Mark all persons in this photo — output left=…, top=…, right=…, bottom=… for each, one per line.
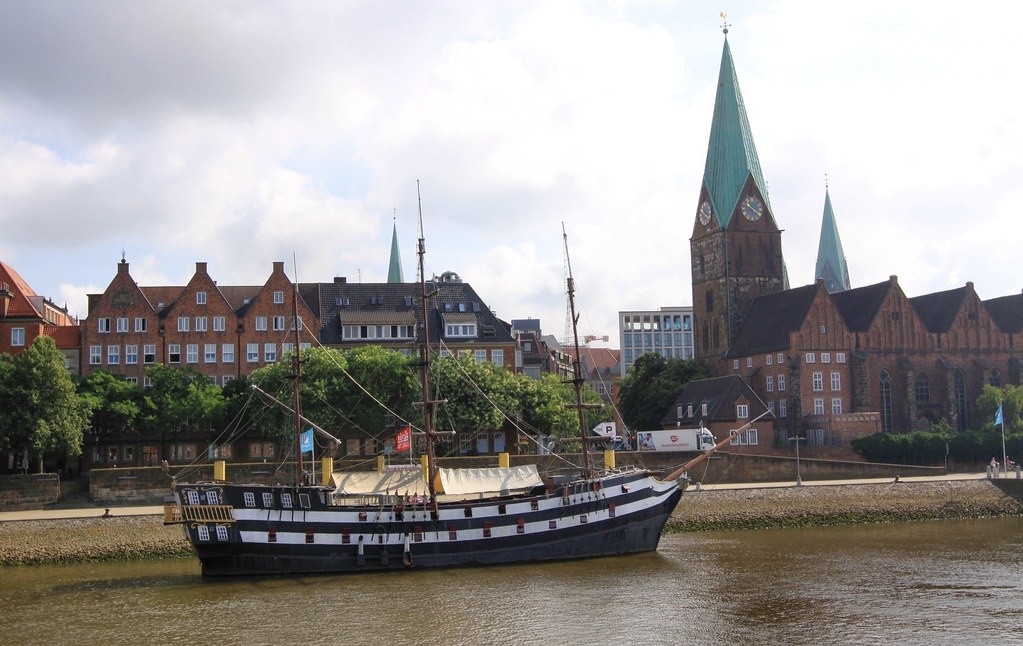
left=594, top=440, right=605, bottom=452
left=1003, top=456, right=1012, bottom=470
left=641, top=437, right=655, bottom=449
left=554, top=440, right=567, bottom=454
left=990, top=458, right=998, bottom=471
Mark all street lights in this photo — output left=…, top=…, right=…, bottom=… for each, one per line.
left=788, top=435, right=806, bottom=485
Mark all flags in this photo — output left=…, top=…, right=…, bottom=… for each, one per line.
left=300, top=428, right=313, bottom=452
left=396, top=428, right=410, bottom=451
left=994, top=405, right=1002, bottom=426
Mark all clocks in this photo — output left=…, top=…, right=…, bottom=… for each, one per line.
left=742, top=196, right=763, bottom=221
left=698, top=202, right=712, bottom=225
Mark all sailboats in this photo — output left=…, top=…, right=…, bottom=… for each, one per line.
left=164, top=178, right=771, bottom=579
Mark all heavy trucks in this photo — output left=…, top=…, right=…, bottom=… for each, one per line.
left=636, top=427, right=716, bottom=452
